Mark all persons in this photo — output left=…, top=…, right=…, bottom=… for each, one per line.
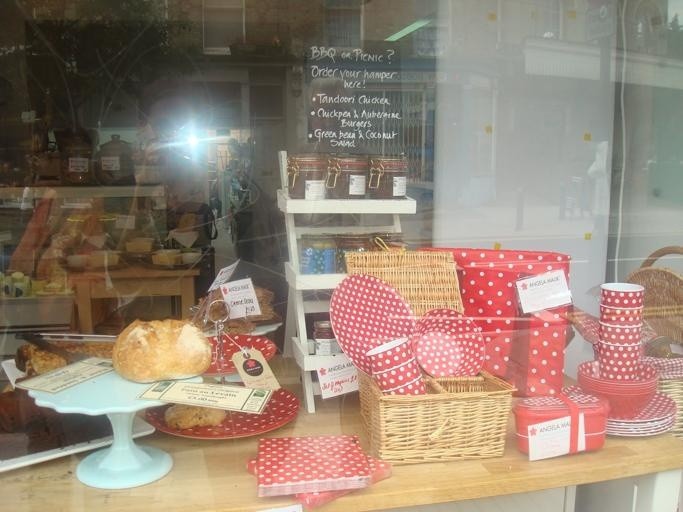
left=288, top=75, right=384, bottom=229
left=102, top=75, right=255, bottom=300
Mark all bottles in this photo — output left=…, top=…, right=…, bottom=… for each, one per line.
left=300, top=232, right=408, bottom=274
left=287, top=154, right=409, bottom=200
left=63, top=136, right=96, bottom=185
left=94, top=134, right=136, bottom=186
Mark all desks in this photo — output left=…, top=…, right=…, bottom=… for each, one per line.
left=2, top=324, right=683, bottom=510
left=72, top=260, right=204, bottom=338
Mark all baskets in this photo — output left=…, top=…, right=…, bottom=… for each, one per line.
left=356, top=367, right=518, bottom=467
left=623, top=245, right=682, bottom=347
left=640, top=356, right=682, bottom=438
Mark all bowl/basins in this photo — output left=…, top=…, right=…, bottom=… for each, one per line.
left=411, top=309, right=487, bottom=381
left=575, top=356, right=664, bottom=419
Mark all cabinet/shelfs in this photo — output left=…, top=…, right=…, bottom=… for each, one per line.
left=275, top=145, right=417, bottom=420
left=2, top=182, right=168, bottom=301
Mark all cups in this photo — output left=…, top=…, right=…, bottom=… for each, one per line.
left=364, top=337, right=427, bottom=398
left=593, top=280, right=648, bottom=385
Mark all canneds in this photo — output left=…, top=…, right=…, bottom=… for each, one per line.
left=301, top=232, right=407, bottom=275
left=312, top=321, right=343, bottom=355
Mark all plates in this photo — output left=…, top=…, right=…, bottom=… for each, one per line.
left=559, top=387, right=677, bottom=438
left=328, top=273, right=418, bottom=379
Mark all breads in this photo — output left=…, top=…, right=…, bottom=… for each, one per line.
left=15, top=285, right=274, bottom=430
